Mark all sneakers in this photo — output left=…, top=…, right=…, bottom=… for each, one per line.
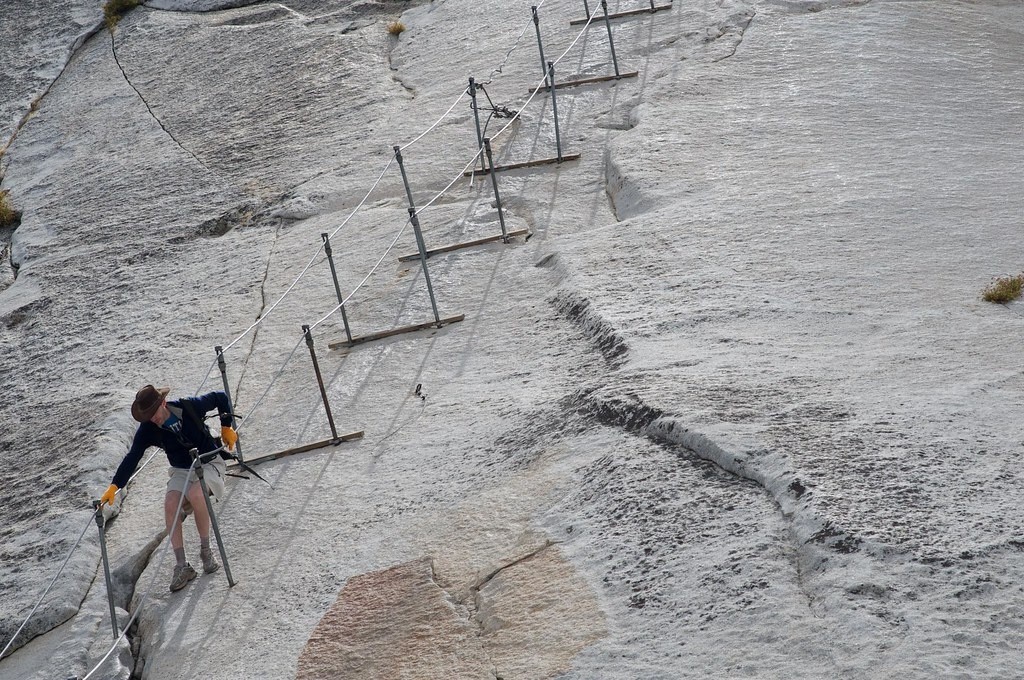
left=170, top=561, right=197, bottom=592
left=199, top=546, right=220, bottom=574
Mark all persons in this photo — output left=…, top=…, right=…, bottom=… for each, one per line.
left=100, top=385, right=238, bottom=592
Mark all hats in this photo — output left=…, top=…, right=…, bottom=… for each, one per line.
left=131, top=385, right=170, bottom=423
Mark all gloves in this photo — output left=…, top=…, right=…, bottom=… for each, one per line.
left=222, top=426, right=238, bottom=450
left=100, top=484, right=118, bottom=510
